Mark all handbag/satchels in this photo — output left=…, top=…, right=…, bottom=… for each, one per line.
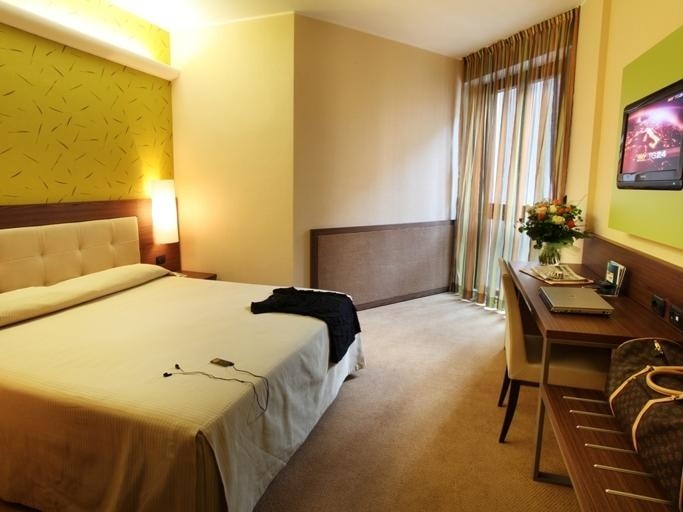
left=606, top=336, right=683, bottom=507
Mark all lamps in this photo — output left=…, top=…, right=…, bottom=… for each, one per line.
left=150, top=179, right=179, bottom=246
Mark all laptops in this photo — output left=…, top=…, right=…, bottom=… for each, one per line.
left=537, top=287, right=616, bottom=316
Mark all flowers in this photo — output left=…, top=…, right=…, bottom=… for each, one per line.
left=513, top=192, right=594, bottom=263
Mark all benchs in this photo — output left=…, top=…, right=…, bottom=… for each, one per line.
left=531, top=385, right=683, bottom=512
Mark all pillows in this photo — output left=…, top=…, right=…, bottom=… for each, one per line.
left=0, top=262, right=174, bottom=330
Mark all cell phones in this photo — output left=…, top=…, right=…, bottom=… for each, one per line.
left=210, top=357, right=234, bottom=367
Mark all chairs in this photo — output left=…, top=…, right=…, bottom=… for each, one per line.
left=496, top=257, right=609, bottom=443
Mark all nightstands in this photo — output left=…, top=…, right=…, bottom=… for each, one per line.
left=173, top=270, right=218, bottom=279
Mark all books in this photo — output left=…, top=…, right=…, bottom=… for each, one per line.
left=520, top=263, right=594, bottom=285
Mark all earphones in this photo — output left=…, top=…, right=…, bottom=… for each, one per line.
left=163, top=372, right=172, bottom=376
left=175, top=363, right=180, bottom=370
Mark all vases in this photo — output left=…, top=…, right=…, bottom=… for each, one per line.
left=539, top=242, right=564, bottom=266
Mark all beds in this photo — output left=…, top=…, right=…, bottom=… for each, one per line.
left=0, top=216, right=364, bottom=512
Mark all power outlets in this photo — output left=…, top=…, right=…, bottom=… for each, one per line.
left=669, top=304, right=683, bottom=328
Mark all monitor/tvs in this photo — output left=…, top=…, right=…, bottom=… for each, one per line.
left=616, top=77, right=683, bottom=191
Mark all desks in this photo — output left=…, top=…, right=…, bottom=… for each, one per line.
left=508, top=261, right=683, bottom=480
